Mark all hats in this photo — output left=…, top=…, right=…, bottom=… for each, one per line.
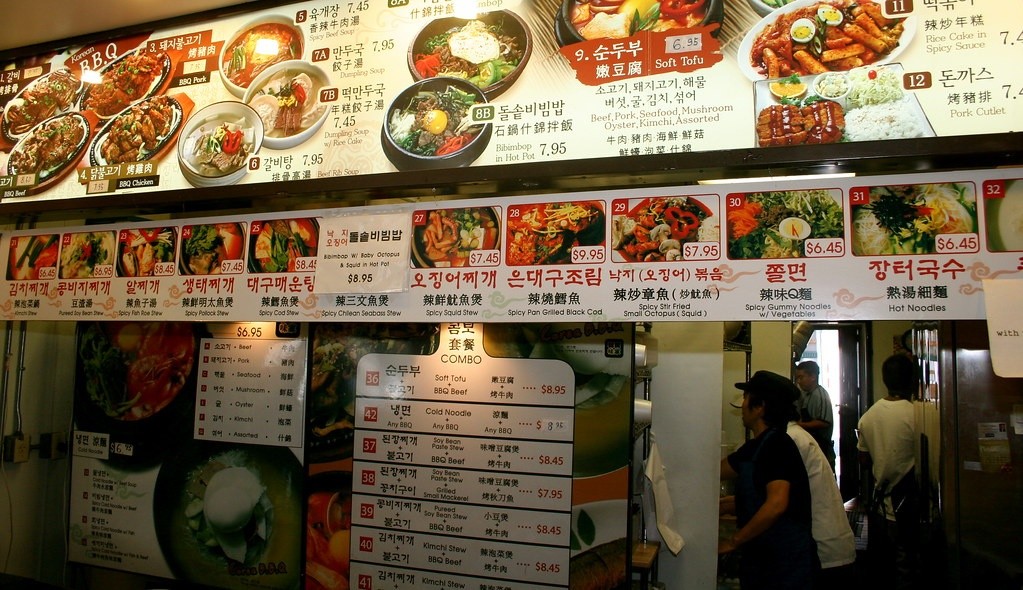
left=735, top=371, right=800, bottom=406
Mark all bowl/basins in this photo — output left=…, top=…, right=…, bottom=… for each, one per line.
left=558, top=0, right=724, bottom=48
left=180, top=222, right=245, bottom=275
left=241, top=60, right=331, bottom=149
left=77, top=321, right=206, bottom=445
left=151, top=442, right=306, bottom=590
left=117, top=227, right=176, bottom=276
left=744, top=0, right=796, bottom=18
left=813, top=71, right=852, bottom=103
left=410, top=207, right=500, bottom=267
left=248, top=217, right=319, bottom=272
left=218, top=13, right=306, bottom=99
left=381, top=77, right=493, bottom=171
left=176, top=101, right=264, bottom=188
left=407, top=8, right=533, bottom=101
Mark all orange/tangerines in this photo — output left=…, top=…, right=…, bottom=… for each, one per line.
left=770, top=83, right=807, bottom=98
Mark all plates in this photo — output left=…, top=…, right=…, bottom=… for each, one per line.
left=7, top=112, right=89, bottom=182
left=737, top=3, right=917, bottom=83
left=89, top=96, right=186, bottom=166
left=1, top=68, right=83, bottom=140
left=80, top=48, right=171, bottom=124
left=752, top=62, right=938, bottom=149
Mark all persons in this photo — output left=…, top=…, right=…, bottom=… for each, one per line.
left=856, top=355, right=915, bottom=590
left=793, top=361, right=835, bottom=474
left=719, top=371, right=856, bottom=590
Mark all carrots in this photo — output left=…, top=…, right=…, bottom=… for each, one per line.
left=726, top=193, right=761, bottom=238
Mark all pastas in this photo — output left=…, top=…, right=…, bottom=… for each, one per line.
left=855, top=185, right=964, bottom=255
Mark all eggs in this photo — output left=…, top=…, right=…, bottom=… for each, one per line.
left=790, top=4, right=844, bottom=43
left=425, top=112, right=448, bottom=135
left=446, top=22, right=500, bottom=64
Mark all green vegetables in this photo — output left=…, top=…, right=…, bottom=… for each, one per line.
left=183, top=226, right=217, bottom=252
left=779, top=94, right=823, bottom=106
left=267, top=229, right=309, bottom=272
left=781, top=75, right=801, bottom=83
left=73, top=233, right=105, bottom=279
left=729, top=191, right=844, bottom=258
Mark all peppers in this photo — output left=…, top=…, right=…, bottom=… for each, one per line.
left=204, top=1, right=730, bottom=155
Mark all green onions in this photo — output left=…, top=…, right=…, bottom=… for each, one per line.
left=813, top=35, right=822, bottom=53
left=22, top=62, right=165, bottom=157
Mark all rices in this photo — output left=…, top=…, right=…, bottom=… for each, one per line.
left=844, top=102, right=924, bottom=142
